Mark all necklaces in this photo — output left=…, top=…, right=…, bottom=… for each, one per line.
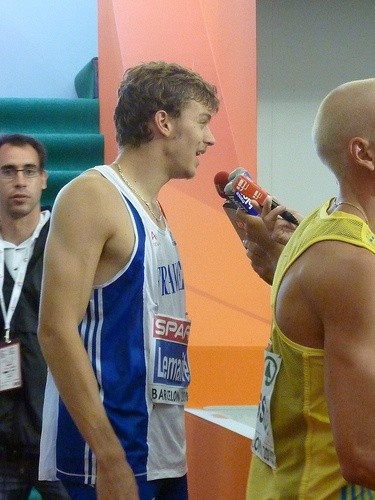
left=111, top=161, right=163, bottom=223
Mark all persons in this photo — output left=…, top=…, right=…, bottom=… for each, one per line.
left=237, top=196, right=303, bottom=285
left=0, top=135, right=52, bottom=500
left=245, top=78, right=375, bottom=500
left=37, top=59, right=220, bottom=500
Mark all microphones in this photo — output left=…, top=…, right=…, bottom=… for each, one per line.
left=213, top=166, right=300, bottom=227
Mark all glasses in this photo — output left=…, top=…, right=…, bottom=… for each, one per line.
left=0, top=167, right=42, bottom=178
